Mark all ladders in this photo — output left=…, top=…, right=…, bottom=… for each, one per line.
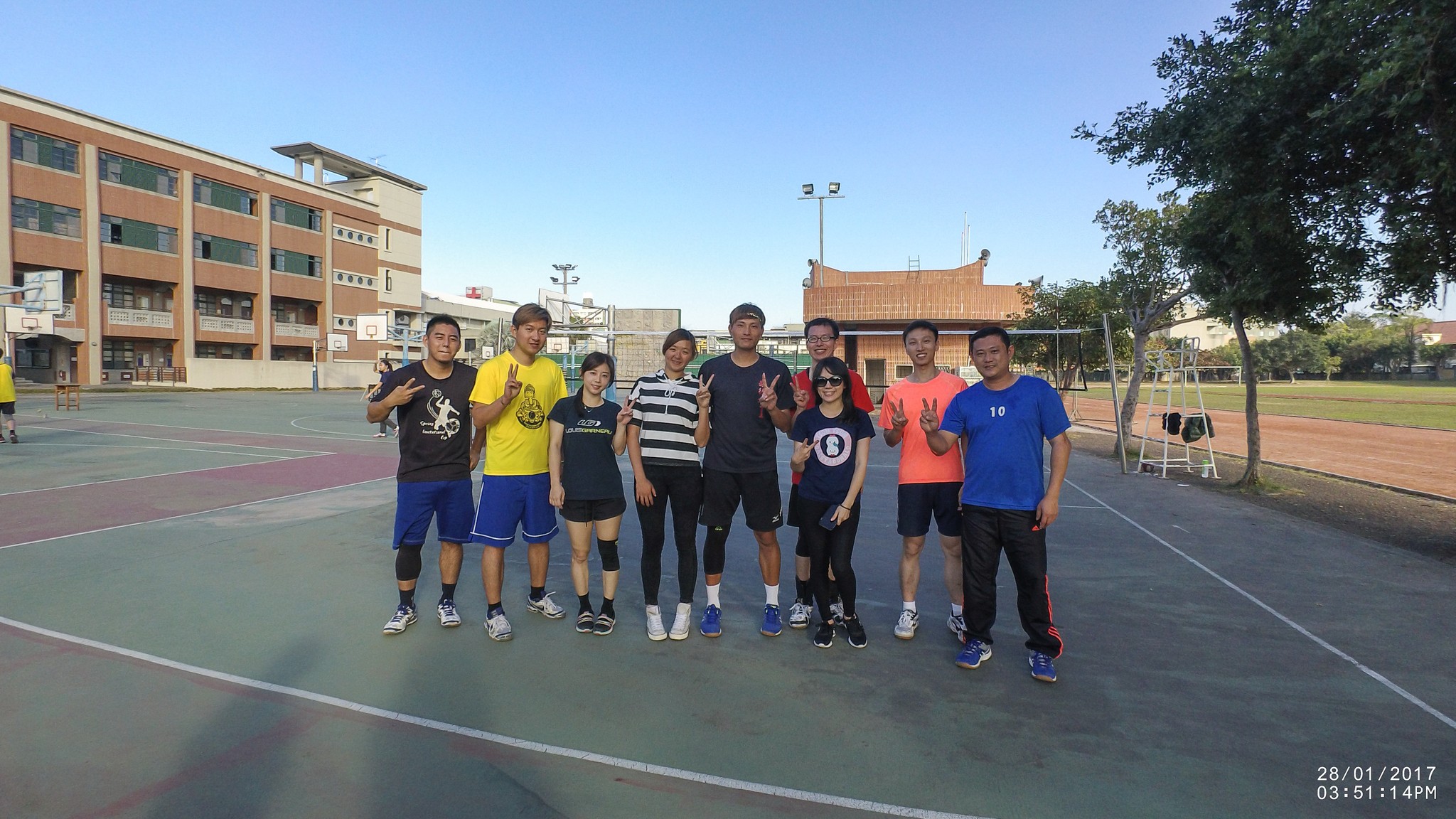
left=1137, top=335, right=1221, bottom=479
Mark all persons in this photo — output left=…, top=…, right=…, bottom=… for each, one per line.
left=469, top=303, right=568, bottom=641
left=366, top=313, right=487, bottom=635
left=548, top=351, right=637, bottom=635
left=626, top=329, right=715, bottom=641
left=365, top=359, right=399, bottom=438
left=919, top=326, right=1072, bottom=681
left=698, top=303, right=797, bottom=637
left=788, top=317, right=970, bottom=647
left=0, top=348, right=20, bottom=444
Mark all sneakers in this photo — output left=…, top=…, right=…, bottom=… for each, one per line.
left=843, top=611, right=867, bottom=648
left=484, top=606, right=513, bottom=642
left=760, top=595, right=782, bottom=636
left=646, top=605, right=667, bottom=641
left=576, top=607, right=595, bottom=633
left=789, top=597, right=814, bottom=629
left=1029, top=650, right=1057, bottom=682
left=955, top=639, right=992, bottom=669
left=829, top=596, right=845, bottom=627
left=383, top=603, right=418, bottom=635
left=813, top=618, right=836, bottom=648
left=526, top=590, right=566, bottom=619
left=437, top=598, right=461, bottom=627
left=669, top=603, right=692, bottom=640
left=593, top=608, right=616, bottom=635
left=894, top=609, right=919, bottom=640
left=700, top=601, right=722, bottom=637
left=947, top=609, right=967, bottom=644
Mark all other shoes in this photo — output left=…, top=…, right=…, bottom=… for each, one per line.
left=9, top=433, right=19, bottom=443
left=373, top=432, right=387, bottom=438
left=0, top=437, right=6, bottom=443
left=393, top=425, right=399, bottom=438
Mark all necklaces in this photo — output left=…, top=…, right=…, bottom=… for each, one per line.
left=584, top=396, right=601, bottom=413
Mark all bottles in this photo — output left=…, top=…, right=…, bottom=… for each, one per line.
left=1201, top=460, right=1209, bottom=478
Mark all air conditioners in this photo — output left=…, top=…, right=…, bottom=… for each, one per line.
left=397, top=315, right=409, bottom=325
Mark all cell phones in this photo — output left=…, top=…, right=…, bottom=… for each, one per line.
left=819, top=504, right=840, bottom=531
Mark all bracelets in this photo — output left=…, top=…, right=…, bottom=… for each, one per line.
left=840, top=503, right=852, bottom=511
left=500, top=396, right=511, bottom=406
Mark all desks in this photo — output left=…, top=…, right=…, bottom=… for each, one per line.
left=368, top=383, right=382, bottom=402
left=53, top=383, right=81, bottom=412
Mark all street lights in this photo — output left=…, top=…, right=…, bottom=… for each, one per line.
left=549, top=264, right=581, bottom=294
left=797, top=182, right=845, bottom=287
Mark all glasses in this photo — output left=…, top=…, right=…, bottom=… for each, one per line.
left=813, top=376, right=843, bottom=387
left=806, top=336, right=837, bottom=343
